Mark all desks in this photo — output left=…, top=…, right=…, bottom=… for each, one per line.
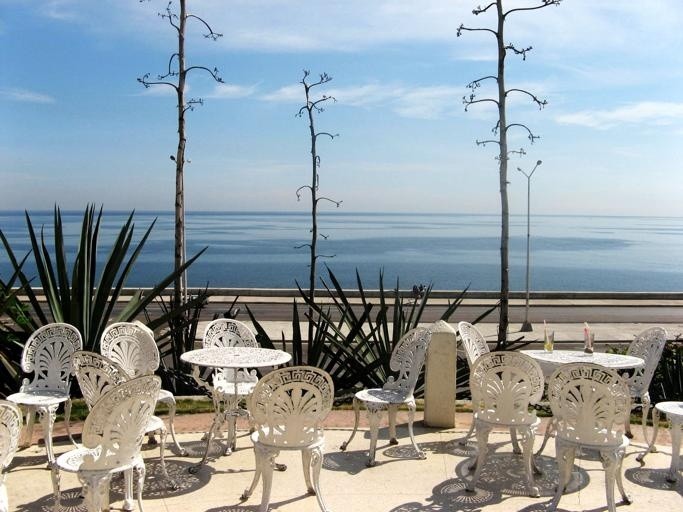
left=180, top=343, right=293, bottom=474
left=518, top=346, right=646, bottom=470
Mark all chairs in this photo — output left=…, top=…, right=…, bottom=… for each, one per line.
left=73, top=349, right=173, bottom=510
left=636, top=400, right=683, bottom=482
left=6, top=321, right=83, bottom=467
left=619, top=327, right=669, bottom=454
left=50, top=372, right=163, bottom=509
left=99, top=320, right=187, bottom=459
left=0, top=398, right=23, bottom=510
left=457, top=320, right=526, bottom=454
left=548, top=362, right=633, bottom=511
left=201, top=317, right=263, bottom=457
left=240, top=365, right=335, bottom=512
left=339, top=326, right=432, bottom=468
left=465, top=350, right=545, bottom=498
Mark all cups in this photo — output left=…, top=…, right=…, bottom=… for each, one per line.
left=543, top=330, right=553, bottom=353
left=584, top=333, right=593, bottom=355
